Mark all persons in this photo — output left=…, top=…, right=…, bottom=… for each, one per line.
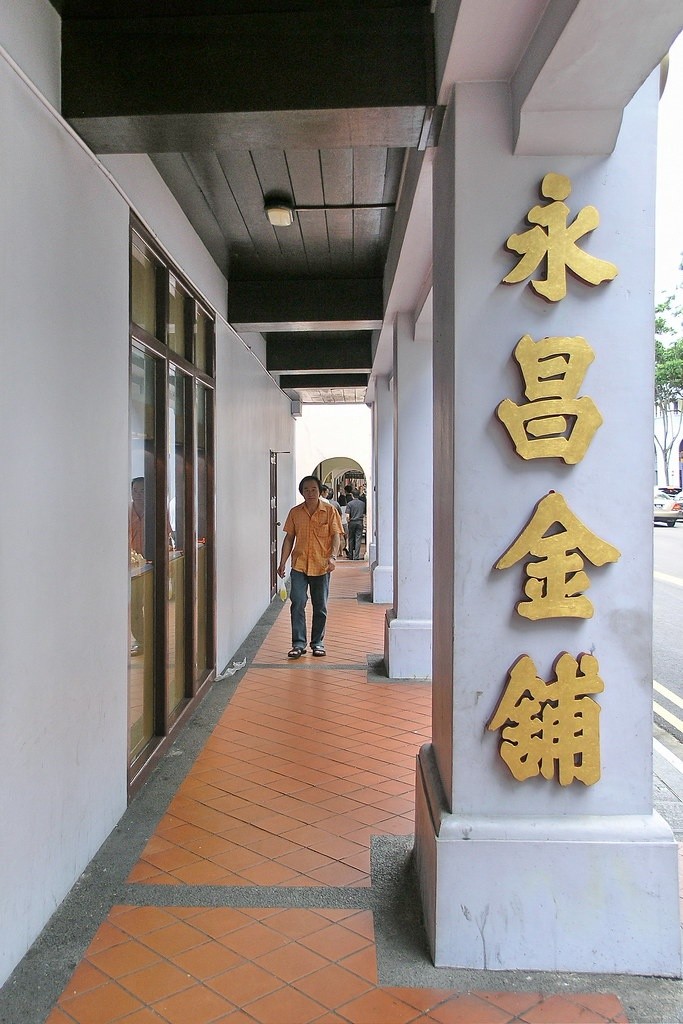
left=318, top=485, right=330, bottom=504
left=338, top=485, right=369, bottom=557
left=277, top=475, right=344, bottom=659
left=326, top=491, right=343, bottom=557
left=128, top=477, right=176, bottom=657
left=345, top=489, right=366, bottom=560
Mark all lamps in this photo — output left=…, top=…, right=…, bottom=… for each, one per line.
left=265, top=205, right=294, bottom=226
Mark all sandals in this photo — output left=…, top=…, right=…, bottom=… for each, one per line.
left=288, top=647, right=306, bottom=659
left=132, top=645, right=143, bottom=657
left=310, top=642, right=326, bottom=656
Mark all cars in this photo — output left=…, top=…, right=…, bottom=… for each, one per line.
left=654, top=485, right=683, bottom=527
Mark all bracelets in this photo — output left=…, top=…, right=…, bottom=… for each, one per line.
left=331, top=555, right=337, bottom=561
